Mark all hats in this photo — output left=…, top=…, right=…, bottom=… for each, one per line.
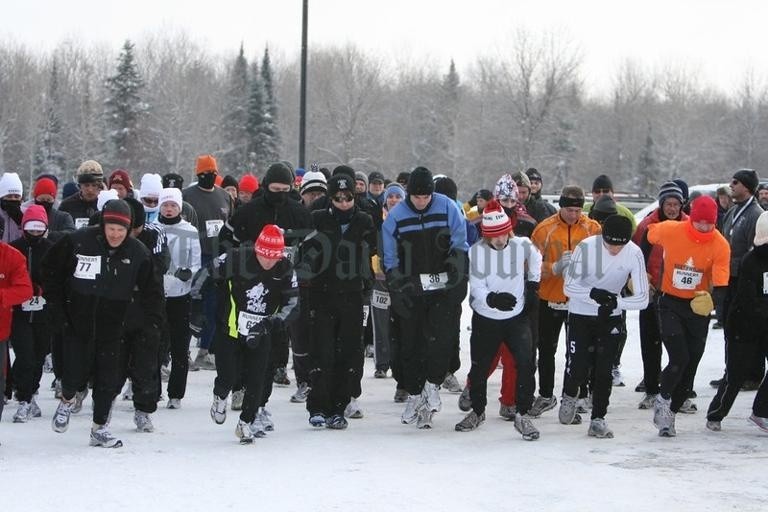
left=109, top=170, right=129, bottom=190
left=214, top=176, right=224, bottom=186
left=591, top=175, right=614, bottom=193
left=408, top=166, right=434, bottom=196
left=384, top=181, right=406, bottom=203
left=264, top=162, right=293, bottom=188
left=77, top=160, right=104, bottom=183
left=601, top=216, right=632, bottom=243
left=397, top=172, right=410, bottom=191
left=354, top=170, right=368, bottom=190
left=733, top=170, right=760, bottom=194
left=692, top=196, right=717, bottom=224
left=432, top=175, right=458, bottom=202
left=319, top=167, right=332, bottom=179
left=366, top=171, right=385, bottom=184
left=560, top=186, right=586, bottom=209
left=0, top=172, right=23, bottom=198
left=300, top=171, right=329, bottom=197
left=327, top=174, right=354, bottom=198
left=753, top=211, right=768, bottom=245
left=591, top=194, right=618, bottom=216
left=480, top=201, right=512, bottom=234
left=23, top=220, right=47, bottom=231
left=124, top=197, right=145, bottom=229
left=759, top=182, right=768, bottom=190
left=332, top=165, right=355, bottom=181
left=139, top=172, right=162, bottom=198
left=220, top=174, right=238, bottom=190
left=672, top=181, right=689, bottom=202
left=197, top=155, right=219, bottom=176
left=97, top=189, right=120, bottom=210
left=238, top=175, right=260, bottom=194
left=476, top=189, right=492, bottom=202
left=103, top=201, right=132, bottom=229
left=63, top=183, right=82, bottom=198
left=34, top=177, right=57, bottom=200
left=157, top=188, right=182, bottom=210
left=510, top=171, right=531, bottom=190
left=494, top=173, right=518, bottom=203
left=716, top=187, right=733, bottom=200
left=659, top=180, right=683, bottom=207
left=525, top=167, right=542, bottom=181
left=254, top=225, right=285, bottom=260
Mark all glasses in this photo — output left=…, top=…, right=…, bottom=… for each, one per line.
left=144, top=198, right=160, bottom=204
left=731, top=180, right=743, bottom=187
left=594, top=190, right=612, bottom=195
left=332, top=194, right=354, bottom=203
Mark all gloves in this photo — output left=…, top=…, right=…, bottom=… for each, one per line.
left=294, top=259, right=314, bottom=282
left=628, top=273, right=656, bottom=302
left=175, top=266, right=192, bottom=281
left=244, top=315, right=279, bottom=354
left=487, top=292, right=516, bottom=310
left=442, top=254, right=465, bottom=290
left=388, top=279, right=413, bottom=320
left=689, top=291, right=715, bottom=317
left=590, top=287, right=619, bottom=315
left=555, top=250, right=571, bottom=275
left=47, top=294, right=71, bottom=326
left=136, top=313, right=161, bottom=342
left=187, top=298, right=211, bottom=338
left=523, top=281, right=540, bottom=304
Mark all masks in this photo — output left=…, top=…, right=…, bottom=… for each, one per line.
left=143, top=205, right=159, bottom=214
left=503, top=206, right=516, bottom=216
left=264, top=189, right=291, bottom=206
left=331, top=206, right=357, bottom=222
left=35, top=200, right=53, bottom=212
left=24, top=231, right=46, bottom=242
left=197, top=173, right=217, bottom=189
left=159, top=213, right=181, bottom=225
left=1, top=200, right=21, bottom=211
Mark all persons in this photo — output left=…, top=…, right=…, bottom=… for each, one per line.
left=525, top=167, right=558, bottom=215
left=309, top=173, right=379, bottom=427
left=710, top=169, right=765, bottom=387
left=636, top=180, right=695, bottom=397
left=162, top=173, right=184, bottom=191
left=191, top=223, right=300, bottom=443
left=0, top=213, right=32, bottom=417
left=211, top=164, right=309, bottom=426
left=393, top=176, right=478, bottom=401
left=461, top=189, right=493, bottom=219
left=299, top=171, right=328, bottom=205
left=455, top=201, right=543, bottom=440
left=8, top=203, right=60, bottom=422
left=532, top=186, right=602, bottom=423
left=30, top=177, right=76, bottom=234
left=582, top=175, right=637, bottom=235
left=137, top=172, right=165, bottom=225
left=108, top=169, right=134, bottom=199
left=308, top=164, right=380, bottom=230
left=217, top=175, right=238, bottom=201
left=559, top=215, right=649, bottom=436
left=153, top=188, right=202, bottom=409
left=1, top=170, right=26, bottom=244
left=381, top=165, right=468, bottom=429
left=58, top=161, right=107, bottom=227
left=509, top=170, right=548, bottom=223
left=707, top=211, right=768, bottom=431
left=87, top=198, right=172, bottom=432
left=639, top=197, right=729, bottom=435
left=588, top=194, right=618, bottom=227
left=369, top=183, right=406, bottom=378
left=238, top=174, right=260, bottom=203
left=182, top=156, right=236, bottom=371
left=367, top=172, right=387, bottom=206
left=631, top=181, right=697, bottom=413
left=36, top=201, right=165, bottom=448
left=60, top=183, right=84, bottom=199
left=709, top=187, right=734, bottom=330
left=354, top=170, right=368, bottom=195
left=753, top=182, right=767, bottom=210
left=456, top=173, right=538, bottom=421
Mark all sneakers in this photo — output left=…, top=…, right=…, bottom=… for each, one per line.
left=416, top=408, right=435, bottom=431
left=291, top=383, right=312, bottom=402
left=612, top=363, right=625, bottom=386
left=375, top=370, right=387, bottom=378
left=710, top=378, right=726, bottom=388
left=194, top=351, right=216, bottom=370
left=185, top=351, right=199, bottom=371
left=44, top=352, right=54, bottom=373
left=458, top=383, right=473, bottom=411
left=678, top=399, right=697, bottom=414
left=654, top=394, right=673, bottom=430
left=160, top=365, right=170, bottom=382
left=52, top=398, right=75, bottom=432
left=636, top=378, right=651, bottom=393
left=587, top=418, right=614, bottom=439
left=236, top=419, right=254, bottom=443
left=500, top=404, right=517, bottom=421
left=707, top=418, right=722, bottom=432
left=326, top=413, right=348, bottom=429
left=395, top=388, right=410, bottom=403
left=71, top=387, right=88, bottom=413
left=740, top=380, right=761, bottom=391
left=273, top=367, right=290, bottom=385
left=528, top=396, right=557, bottom=416
left=13, top=396, right=41, bottom=422
left=658, top=416, right=676, bottom=437
left=345, top=398, right=363, bottom=417
left=401, top=389, right=429, bottom=424
left=50, top=377, right=65, bottom=399
left=442, top=374, right=462, bottom=392
left=251, top=417, right=265, bottom=438
left=421, top=379, right=444, bottom=413
left=88, top=425, right=123, bottom=448
left=134, top=410, right=154, bottom=432
left=640, top=394, right=657, bottom=410
left=559, top=383, right=582, bottom=424
left=364, top=344, right=376, bottom=357
left=211, top=395, right=226, bottom=424
left=123, top=381, right=134, bottom=400
left=713, top=322, right=723, bottom=330
left=231, top=388, right=244, bottom=410
left=455, top=411, right=486, bottom=431
left=748, top=414, right=768, bottom=432
left=258, top=409, right=272, bottom=431
left=167, top=398, right=182, bottom=409
left=309, top=409, right=326, bottom=427
left=515, top=413, right=540, bottom=440
